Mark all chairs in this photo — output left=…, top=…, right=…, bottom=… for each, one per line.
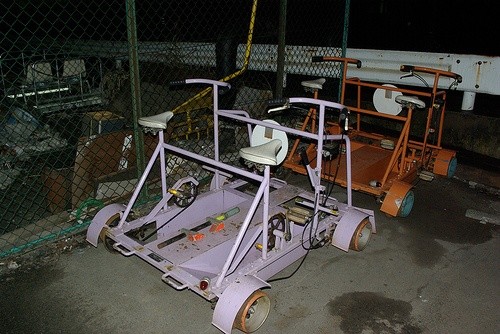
left=25, top=59, right=91, bottom=94
